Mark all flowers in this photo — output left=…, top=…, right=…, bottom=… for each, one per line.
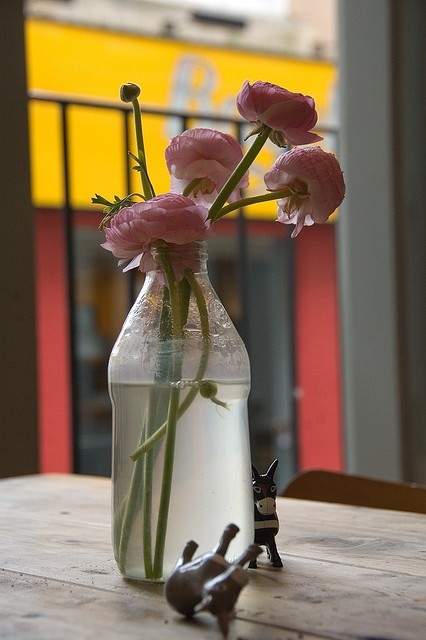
left=90, top=79, right=348, bottom=582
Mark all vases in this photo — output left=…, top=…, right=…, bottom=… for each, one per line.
left=107, top=239, right=257, bottom=583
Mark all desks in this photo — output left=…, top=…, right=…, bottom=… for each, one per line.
left=0, top=472, right=424, bottom=639
